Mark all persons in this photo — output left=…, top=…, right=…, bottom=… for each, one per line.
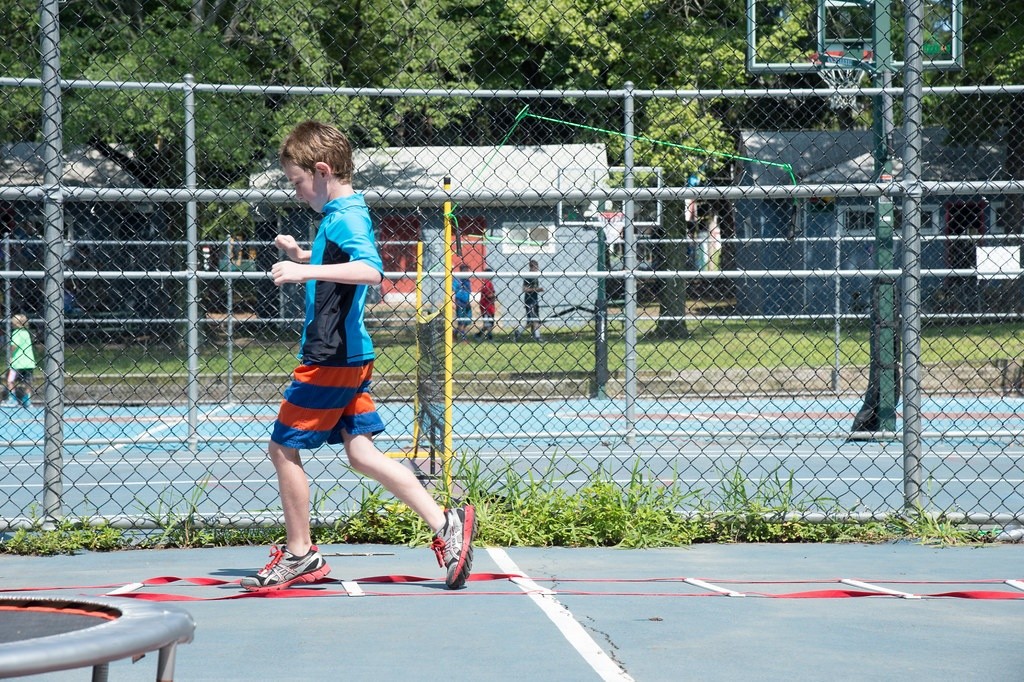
left=474, top=269, right=495, bottom=343
left=451, top=264, right=472, bottom=343
left=513, top=260, right=547, bottom=343
left=239, top=120, right=477, bottom=593
left=1, top=313, right=36, bottom=408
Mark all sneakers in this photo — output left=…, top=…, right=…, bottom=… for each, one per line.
left=239, top=544, right=331, bottom=591
left=429, top=505, right=477, bottom=589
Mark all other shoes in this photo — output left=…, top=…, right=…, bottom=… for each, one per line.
left=1, top=400, right=19, bottom=407
left=486, top=332, right=495, bottom=342
left=473, top=333, right=482, bottom=342
left=532, top=336, right=544, bottom=343
left=17, top=398, right=33, bottom=408
left=454, top=327, right=466, bottom=339
left=515, top=330, right=522, bottom=342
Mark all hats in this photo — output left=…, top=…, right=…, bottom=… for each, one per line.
left=12, top=314, right=29, bottom=329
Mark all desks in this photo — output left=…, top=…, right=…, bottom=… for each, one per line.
left=523, top=299, right=626, bottom=338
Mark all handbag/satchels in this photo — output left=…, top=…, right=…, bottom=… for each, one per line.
left=7, top=369, right=17, bottom=392
